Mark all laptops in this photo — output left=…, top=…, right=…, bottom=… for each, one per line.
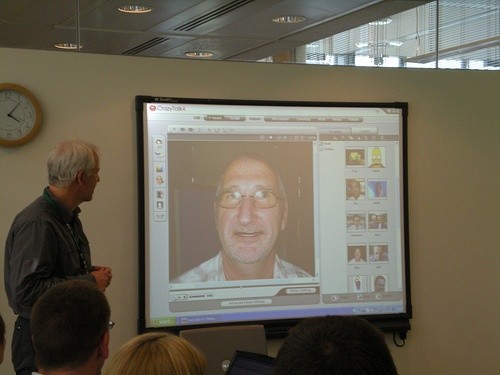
left=179, top=325, right=268, bottom=375
left=224, top=350, right=276, bottom=375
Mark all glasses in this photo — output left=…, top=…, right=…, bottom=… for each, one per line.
left=106, top=320, right=115, bottom=329
left=212, top=188, right=288, bottom=209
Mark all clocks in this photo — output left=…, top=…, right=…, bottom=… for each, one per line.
left=0, top=83, right=43, bottom=147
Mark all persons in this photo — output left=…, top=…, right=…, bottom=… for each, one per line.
left=369, top=245, right=388, bottom=261
left=27, top=279, right=117, bottom=375
left=368, top=214, right=387, bottom=229
left=107, top=330, right=208, bottom=375
left=169, top=155, right=314, bottom=284
left=368, top=181, right=386, bottom=197
left=275, top=316, right=399, bottom=375
left=346, top=180, right=366, bottom=199
left=348, top=246, right=366, bottom=262
left=0, top=141, right=113, bottom=375
left=348, top=214, right=365, bottom=230
left=371, top=275, right=388, bottom=292
